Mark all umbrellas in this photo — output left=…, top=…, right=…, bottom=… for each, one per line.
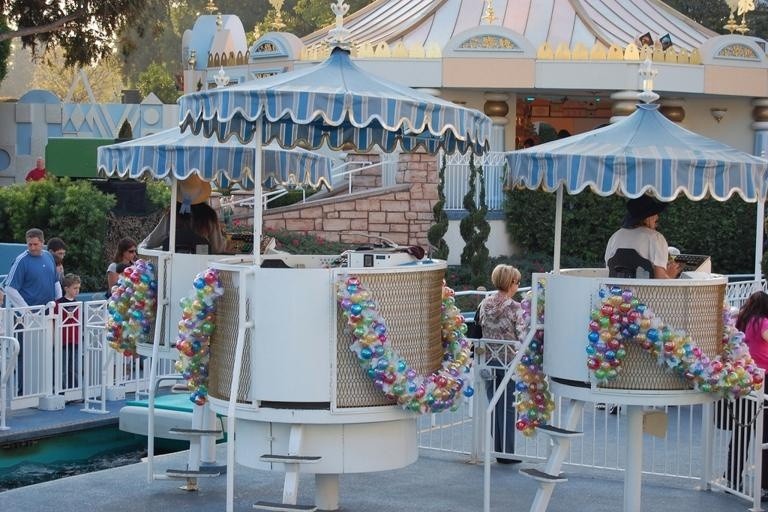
left=177, top=48, right=492, bottom=266
left=503, top=89, right=768, bottom=276
left=94, top=120, right=336, bottom=255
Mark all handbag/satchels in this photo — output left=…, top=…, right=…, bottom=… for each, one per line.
left=468, top=309, right=483, bottom=358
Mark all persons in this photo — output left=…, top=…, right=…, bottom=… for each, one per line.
left=475, top=263, right=524, bottom=466
left=18, top=154, right=49, bottom=182
left=106, top=236, right=137, bottom=386
left=719, top=290, right=768, bottom=495
left=603, top=198, right=687, bottom=280
left=1, top=229, right=83, bottom=395
left=141, top=175, right=231, bottom=255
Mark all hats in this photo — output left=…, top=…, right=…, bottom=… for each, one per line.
left=177, top=174, right=212, bottom=205
left=623, top=194, right=665, bottom=227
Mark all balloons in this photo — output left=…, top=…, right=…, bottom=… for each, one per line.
left=105, top=258, right=158, bottom=362
left=515, top=279, right=557, bottom=439
left=335, top=276, right=476, bottom=413
left=172, top=266, right=226, bottom=407
left=585, top=286, right=764, bottom=404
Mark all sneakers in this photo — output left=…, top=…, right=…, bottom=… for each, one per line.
left=610, top=404, right=621, bottom=414
left=595, top=402, right=605, bottom=409
